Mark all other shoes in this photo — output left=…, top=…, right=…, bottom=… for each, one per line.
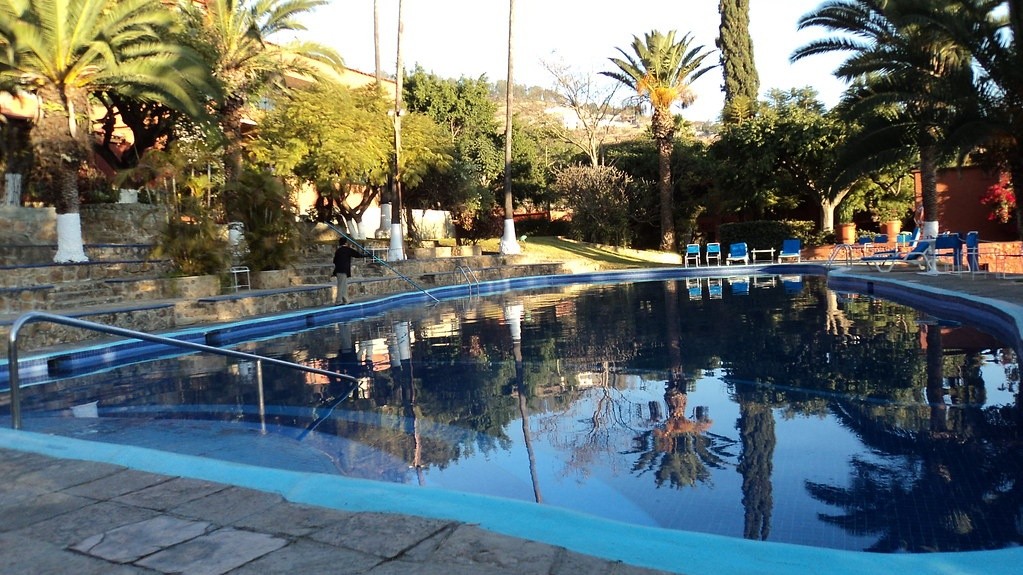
left=349, top=303, right=355, bottom=304
left=335, top=304, right=345, bottom=306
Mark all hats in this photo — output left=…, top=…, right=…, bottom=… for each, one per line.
left=339, top=237, right=347, bottom=246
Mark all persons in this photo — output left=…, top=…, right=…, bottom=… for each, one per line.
left=332, top=237, right=361, bottom=306
left=338, top=321, right=361, bottom=377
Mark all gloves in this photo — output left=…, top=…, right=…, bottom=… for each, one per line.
left=364, top=253, right=373, bottom=257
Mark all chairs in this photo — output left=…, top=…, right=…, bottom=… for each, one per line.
left=706, top=243, right=721, bottom=267
left=684, top=244, right=701, bottom=267
left=778, top=240, right=800, bottom=263
left=858, top=228, right=979, bottom=272
left=726, top=243, right=749, bottom=267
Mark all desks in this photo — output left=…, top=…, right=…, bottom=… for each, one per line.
left=218, top=266, right=251, bottom=293
left=995, top=254, right=1023, bottom=280
left=751, top=247, right=776, bottom=262
left=364, top=245, right=390, bottom=263
